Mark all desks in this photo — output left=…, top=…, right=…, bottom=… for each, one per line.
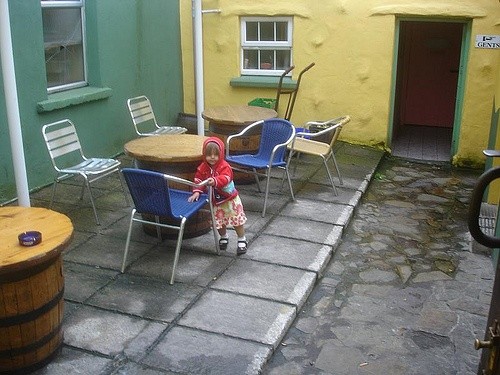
left=0, top=206, right=75, bottom=375
left=202, top=105, right=278, bottom=184
left=124, top=134, right=215, bottom=239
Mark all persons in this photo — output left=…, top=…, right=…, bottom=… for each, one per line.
left=188, top=137, right=248, bottom=253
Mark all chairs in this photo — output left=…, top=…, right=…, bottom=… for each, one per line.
left=43, top=96, right=351, bottom=285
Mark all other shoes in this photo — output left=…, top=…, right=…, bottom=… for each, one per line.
left=237, top=239, right=247, bottom=254
left=219, top=238, right=229, bottom=249
